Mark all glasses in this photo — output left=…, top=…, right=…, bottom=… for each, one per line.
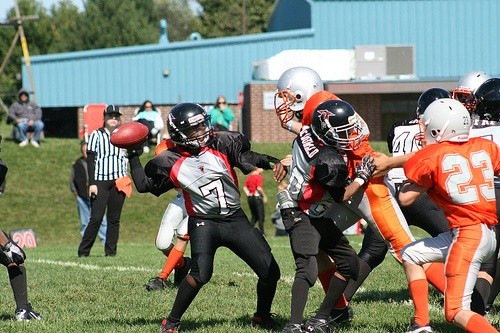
left=145, top=106, right=152, bottom=108
left=218, top=102, right=226, bottom=104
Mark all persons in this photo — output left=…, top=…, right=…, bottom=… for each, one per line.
left=0, top=135, right=42, bottom=322
left=273, top=67, right=500, bottom=333
left=78, top=104, right=129, bottom=256
left=68, top=141, right=110, bottom=246
left=8, top=91, right=45, bottom=146
left=243, top=167, right=269, bottom=237
left=110, top=102, right=286, bottom=333
left=144, top=182, right=193, bottom=291
left=136, top=101, right=163, bottom=154
left=208, top=96, right=233, bottom=133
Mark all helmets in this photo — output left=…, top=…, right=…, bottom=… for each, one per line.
left=167, top=103, right=213, bottom=149
left=416, top=88, right=452, bottom=123
left=278, top=67, right=323, bottom=112
left=311, top=100, right=362, bottom=150
left=453, top=71, right=492, bottom=114
left=419, top=98, right=471, bottom=147
left=466, top=77, right=500, bottom=122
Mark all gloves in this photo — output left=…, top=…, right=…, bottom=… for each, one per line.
left=354, top=153, right=377, bottom=184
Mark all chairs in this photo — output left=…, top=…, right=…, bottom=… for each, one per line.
left=82, top=104, right=107, bottom=136
left=134, top=107, right=162, bottom=145
left=204, top=106, right=232, bottom=132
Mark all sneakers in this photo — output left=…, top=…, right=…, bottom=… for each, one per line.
left=252, top=312, right=281, bottom=331
left=15, top=303, right=43, bottom=322
left=161, top=318, right=181, bottom=333
left=174, top=257, right=192, bottom=288
left=304, top=316, right=331, bottom=333
left=282, top=322, right=311, bottom=333
left=143, top=276, right=171, bottom=292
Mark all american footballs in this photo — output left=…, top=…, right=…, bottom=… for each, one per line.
left=108, top=122, right=150, bottom=148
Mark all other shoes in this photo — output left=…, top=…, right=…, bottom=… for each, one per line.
left=151, top=128, right=159, bottom=136
left=143, top=146, right=150, bottom=153
left=485, top=304, right=496, bottom=316
left=403, top=316, right=432, bottom=333
left=30, top=140, right=39, bottom=148
left=328, top=306, right=352, bottom=323
left=432, top=289, right=444, bottom=307
left=19, top=139, right=28, bottom=147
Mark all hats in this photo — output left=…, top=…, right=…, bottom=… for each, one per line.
left=103, top=105, right=122, bottom=116
